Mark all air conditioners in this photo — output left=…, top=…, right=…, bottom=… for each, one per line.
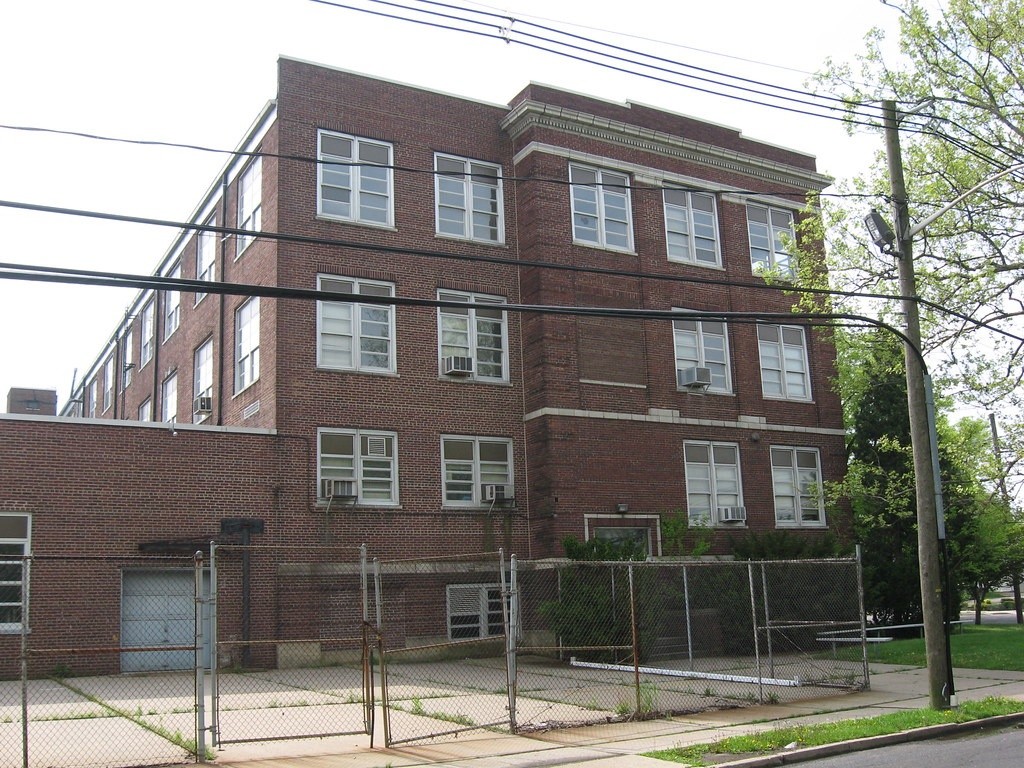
left=720, top=506, right=746, bottom=523
left=486, top=485, right=514, bottom=499
left=442, top=355, right=474, bottom=375
left=193, top=396, right=211, bottom=414
left=682, top=365, right=712, bottom=385
left=326, top=479, right=359, bottom=496
